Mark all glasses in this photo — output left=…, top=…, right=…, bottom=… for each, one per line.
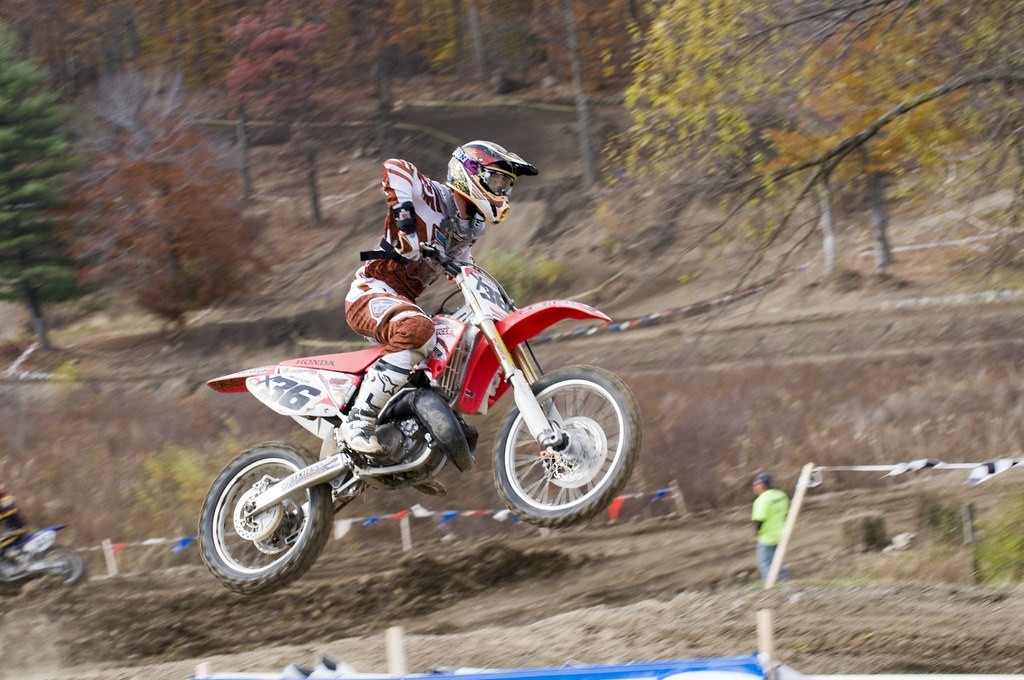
left=452, top=146, right=517, bottom=197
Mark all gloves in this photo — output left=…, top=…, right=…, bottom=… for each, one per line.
left=394, top=231, right=420, bottom=261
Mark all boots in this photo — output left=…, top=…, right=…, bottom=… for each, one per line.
left=338, top=360, right=410, bottom=453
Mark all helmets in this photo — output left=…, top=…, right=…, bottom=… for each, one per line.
left=447, top=140, right=538, bottom=225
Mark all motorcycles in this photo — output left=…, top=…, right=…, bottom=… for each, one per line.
left=198, top=238, right=644, bottom=594
left=0, top=524, right=84, bottom=595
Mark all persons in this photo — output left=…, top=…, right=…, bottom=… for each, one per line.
left=749, top=472, right=789, bottom=585
left=0, top=481, right=30, bottom=566
left=334, top=141, right=538, bottom=497
